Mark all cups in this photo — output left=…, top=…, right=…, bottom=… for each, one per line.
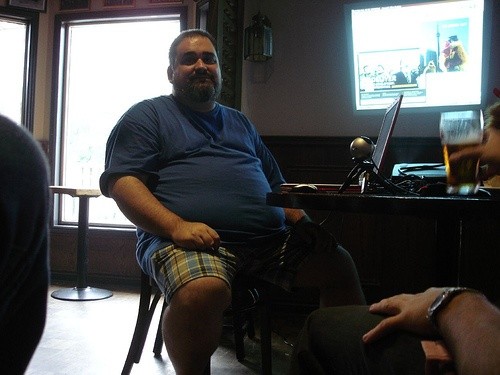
left=441, top=110, right=484, bottom=193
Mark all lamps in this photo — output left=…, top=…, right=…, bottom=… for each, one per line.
left=244, top=10, right=273, bottom=62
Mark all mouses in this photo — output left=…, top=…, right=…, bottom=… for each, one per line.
left=293, top=184, right=318, bottom=193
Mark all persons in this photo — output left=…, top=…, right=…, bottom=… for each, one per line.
left=99, top=28, right=363, bottom=375
left=362, top=57, right=418, bottom=89
left=292, top=126, right=500, bottom=375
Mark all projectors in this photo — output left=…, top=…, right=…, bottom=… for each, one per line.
left=392, top=162, right=448, bottom=194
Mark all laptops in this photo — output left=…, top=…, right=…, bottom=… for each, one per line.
left=280, top=94, right=403, bottom=192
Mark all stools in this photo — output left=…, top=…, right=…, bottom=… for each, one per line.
left=121, top=259, right=275, bottom=375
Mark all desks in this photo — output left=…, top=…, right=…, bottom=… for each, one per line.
left=49, top=186, right=114, bottom=301
left=265, top=192, right=500, bottom=290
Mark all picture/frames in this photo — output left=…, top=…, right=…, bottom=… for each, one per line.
left=7, top=0, right=48, bottom=14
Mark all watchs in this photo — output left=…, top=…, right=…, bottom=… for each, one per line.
left=428, top=286, right=477, bottom=340
